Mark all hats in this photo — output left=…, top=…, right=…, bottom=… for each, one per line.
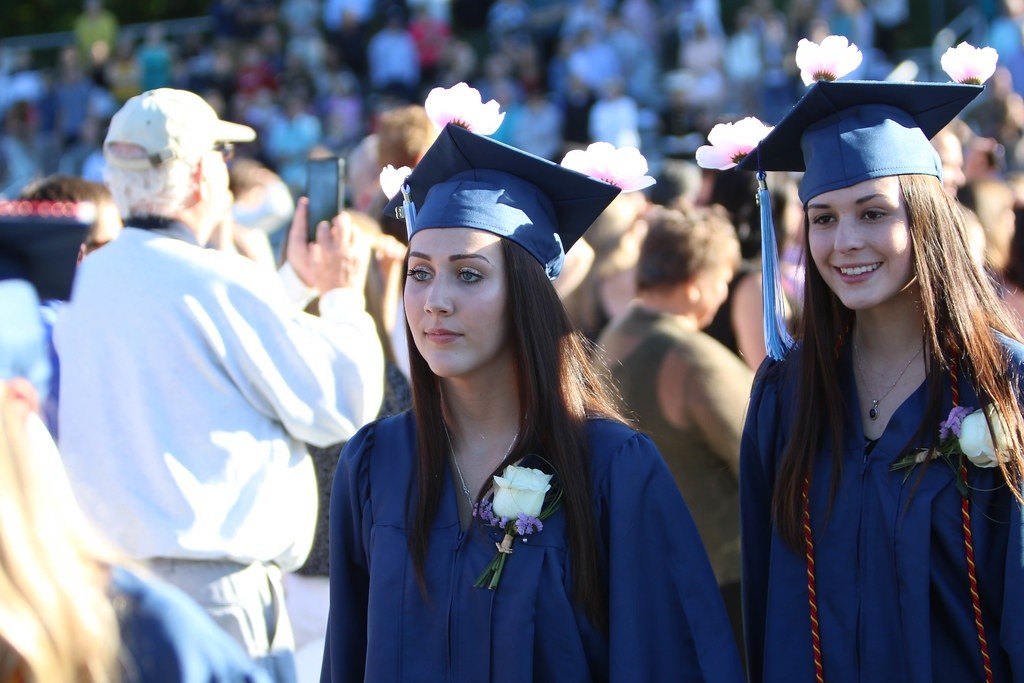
left=733, top=81, right=985, bottom=360
left=103, top=87, right=257, bottom=171
left=379, top=121, right=621, bottom=280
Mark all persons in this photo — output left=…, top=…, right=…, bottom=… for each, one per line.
left=316, top=80, right=748, bottom=683
left=1, top=0, right=1023, bottom=683
left=695, top=32, right=1024, bottom=683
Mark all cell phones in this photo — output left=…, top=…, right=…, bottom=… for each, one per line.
left=307, top=157, right=345, bottom=242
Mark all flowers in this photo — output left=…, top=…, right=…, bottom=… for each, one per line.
left=379, top=164, right=413, bottom=201
left=471, top=463, right=562, bottom=590
left=941, top=41, right=999, bottom=85
left=560, top=141, right=657, bottom=193
left=425, top=82, right=507, bottom=135
left=888, top=403, right=1015, bottom=500
left=795, top=35, right=862, bottom=81
left=695, top=118, right=775, bottom=171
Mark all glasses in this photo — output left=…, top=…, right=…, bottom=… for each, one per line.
left=198, top=142, right=235, bottom=164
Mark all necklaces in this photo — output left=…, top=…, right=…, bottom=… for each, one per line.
left=852, top=332, right=924, bottom=421
left=440, top=417, right=523, bottom=511
